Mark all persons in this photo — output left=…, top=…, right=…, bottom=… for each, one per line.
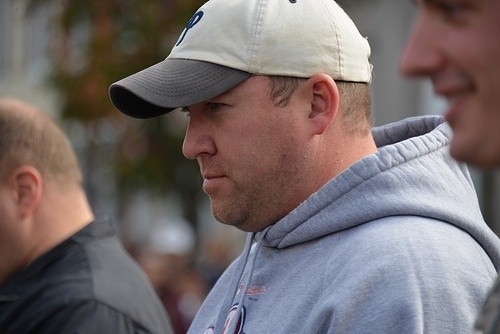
left=109, top=0, right=499, bottom=333
left=2, top=98, right=178, bottom=332
left=401, top=1, right=499, bottom=334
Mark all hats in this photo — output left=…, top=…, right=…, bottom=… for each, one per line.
left=108, top=1, right=375, bottom=122
